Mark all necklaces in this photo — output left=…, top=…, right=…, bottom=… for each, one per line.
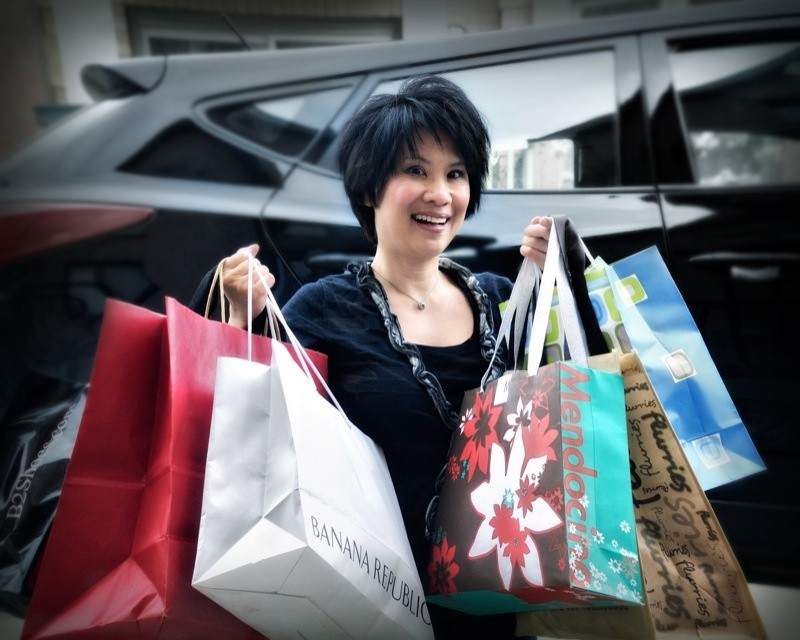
left=371, top=266, right=440, bottom=310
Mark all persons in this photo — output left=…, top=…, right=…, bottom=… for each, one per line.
left=223, top=77, right=552, bottom=640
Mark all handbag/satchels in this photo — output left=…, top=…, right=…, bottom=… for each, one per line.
left=18, top=257, right=329, bottom=640
left=515, top=217, right=769, bottom=640
left=423, top=217, right=645, bottom=610
left=498, top=217, right=766, bottom=492
left=191, top=246, right=433, bottom=640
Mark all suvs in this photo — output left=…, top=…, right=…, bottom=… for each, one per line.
left=2, top=0, right=800, bottom=587
